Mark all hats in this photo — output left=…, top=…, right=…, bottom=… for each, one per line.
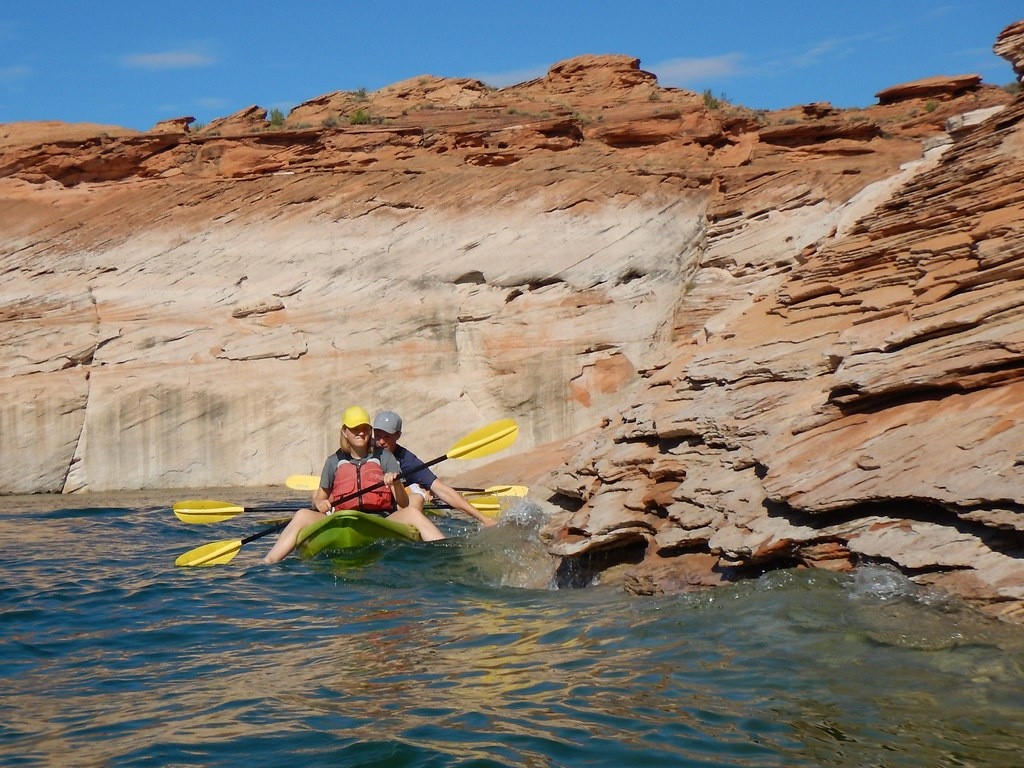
left=374, top=410, right=402, bottom=434
left=342, top=406, right=373, bottom=429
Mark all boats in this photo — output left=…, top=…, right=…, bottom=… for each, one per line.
left=295, top=502, right=444, bottom=568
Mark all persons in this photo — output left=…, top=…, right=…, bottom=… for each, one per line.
left=373, top=412, right=492, bottom=522
left=265, top=407, right=445, bottom=562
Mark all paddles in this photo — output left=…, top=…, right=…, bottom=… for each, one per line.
left=284, top=470, right=530, bottom=498
left=174, top=419, right=519, bottom=569
left=174, top=494, right=503, bottom=526
